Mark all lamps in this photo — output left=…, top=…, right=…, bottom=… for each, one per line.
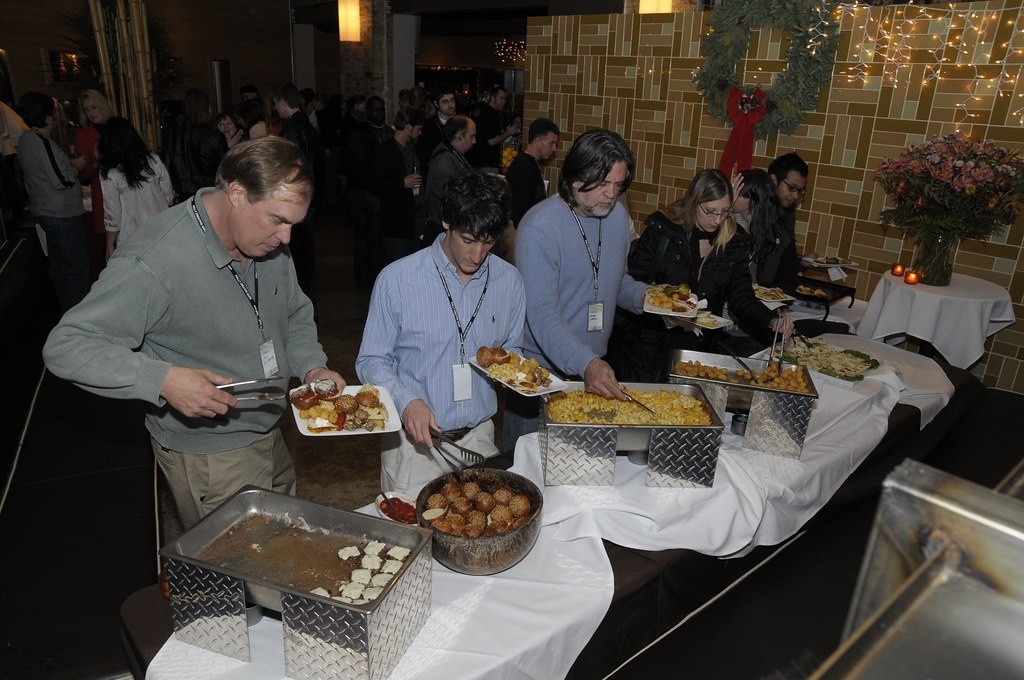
left=337, top=0, right=361, bottom=42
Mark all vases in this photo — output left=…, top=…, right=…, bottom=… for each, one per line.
left=916, top=234, right=960, bottom=286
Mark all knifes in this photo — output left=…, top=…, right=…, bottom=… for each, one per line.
left=719, top=342, right=758, bottom=385
left=621, top=391, right=655, bottom=416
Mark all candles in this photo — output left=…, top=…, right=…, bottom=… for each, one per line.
left=904, top=271, right=918, bottom=285
left=891, top=264, right=905, bottom=276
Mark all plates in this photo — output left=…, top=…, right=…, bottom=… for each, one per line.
left=643, top=292, right=698, bottom=318
left=802, top=257, right=859, bottom=267
left=290, top=385, right=401, bottom=436
left=469, top=351, right=568, bottom=397
left=752, top=286, right=795, bottom=302
left=675, top=310, right=733, bottom=329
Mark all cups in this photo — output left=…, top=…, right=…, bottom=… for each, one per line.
left=904, top=271, right=919, bottom=284
left=892, top=263, right=906, bottom=276
left=82, top=186, right=92, bottom=211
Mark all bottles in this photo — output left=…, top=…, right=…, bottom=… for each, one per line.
left=410, top=167, right=426, bottom=206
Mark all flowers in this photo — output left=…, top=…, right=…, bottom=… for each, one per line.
left=874, top=133, right=1024, bottom=238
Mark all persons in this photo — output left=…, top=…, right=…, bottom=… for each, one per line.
left=16, top=87, right=173, bottom=265
left=502, top=128, right=677, bottom=453
left=355, top=174, right=525, bottom=501
left=198, top=82, right=522, bottom=326
left=506, top=118, right=559, bottom=231
left=768, top=152, right=819, bottom=293
left=41, top=136, right=345, bottom=612
left=629, top=161, right=793, bottom=382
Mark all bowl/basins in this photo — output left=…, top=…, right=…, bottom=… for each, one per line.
left=417, top=469, right=543, bottom=576
left=375, top=492, right=420, bottom=527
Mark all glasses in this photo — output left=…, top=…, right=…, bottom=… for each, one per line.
left=368, top=107, right=386, bottom=112
left=780, top=179, right=807, bottom=195
left=698, top=203, right=736, bottom=219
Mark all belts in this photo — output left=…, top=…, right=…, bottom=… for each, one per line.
left=442, top=427, right=472, bottom=444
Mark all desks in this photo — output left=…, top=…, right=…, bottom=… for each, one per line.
left=752, top=297, right=905, bottom=346
left=858, top=268, right=1016, bottom=372
left=120, top=331, right=990, bottom=680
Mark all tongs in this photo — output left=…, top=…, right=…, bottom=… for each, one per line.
left=766, top=313, right=786, bottom=377
left=429, top=425, right=485, bottom=476
left=214, top=376, right=285, bottom=400
